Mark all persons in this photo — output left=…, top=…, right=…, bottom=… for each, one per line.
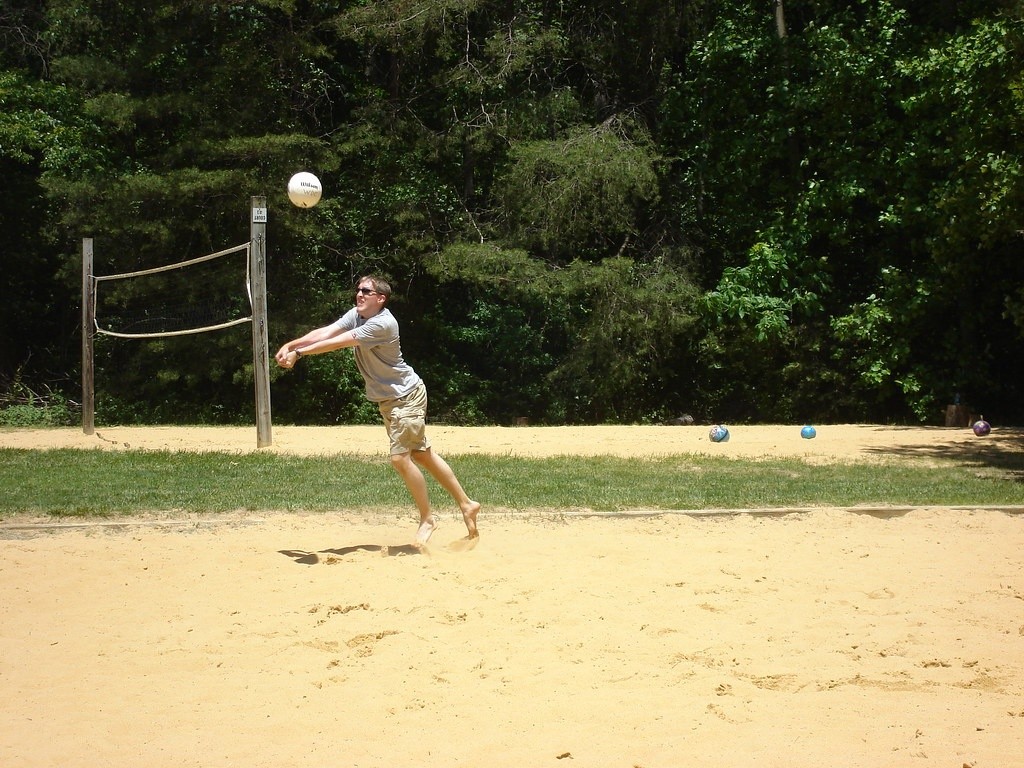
left=274, top=273, right=484, bottom=553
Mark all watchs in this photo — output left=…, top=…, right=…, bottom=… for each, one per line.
left=294, top=348, right=303, bottom=360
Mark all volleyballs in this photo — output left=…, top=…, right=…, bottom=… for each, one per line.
left=709, top=425, right=730, bottom=443
left=800, top=425, right=816, bottom=439
left=973, top=421, right=991, bottom=437
left=286, top=172, right=322, bottom=208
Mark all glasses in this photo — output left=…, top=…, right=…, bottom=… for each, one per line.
left=356, top=287, right=384, bottom=296
